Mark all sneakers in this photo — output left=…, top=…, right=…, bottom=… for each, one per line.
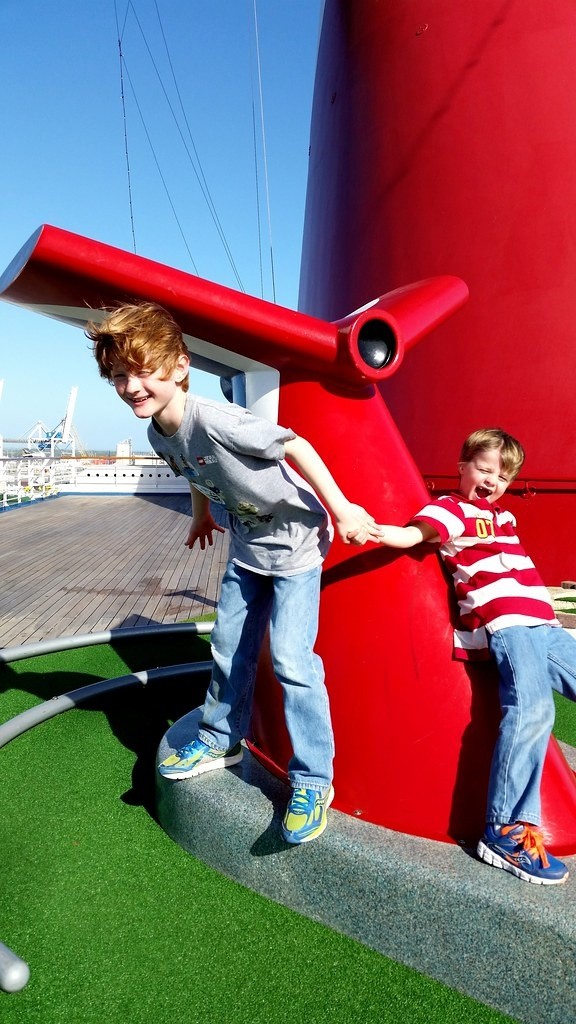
left=280, top=782, right=335, bottom=844
left=477, top=821, right=570, bottom=885
left=157, top=734, right=244, bottom=780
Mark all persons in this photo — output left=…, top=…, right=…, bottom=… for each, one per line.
left=348, top=428, right=576, bottom=885
left=82, top=296, right=384, bottom=843
